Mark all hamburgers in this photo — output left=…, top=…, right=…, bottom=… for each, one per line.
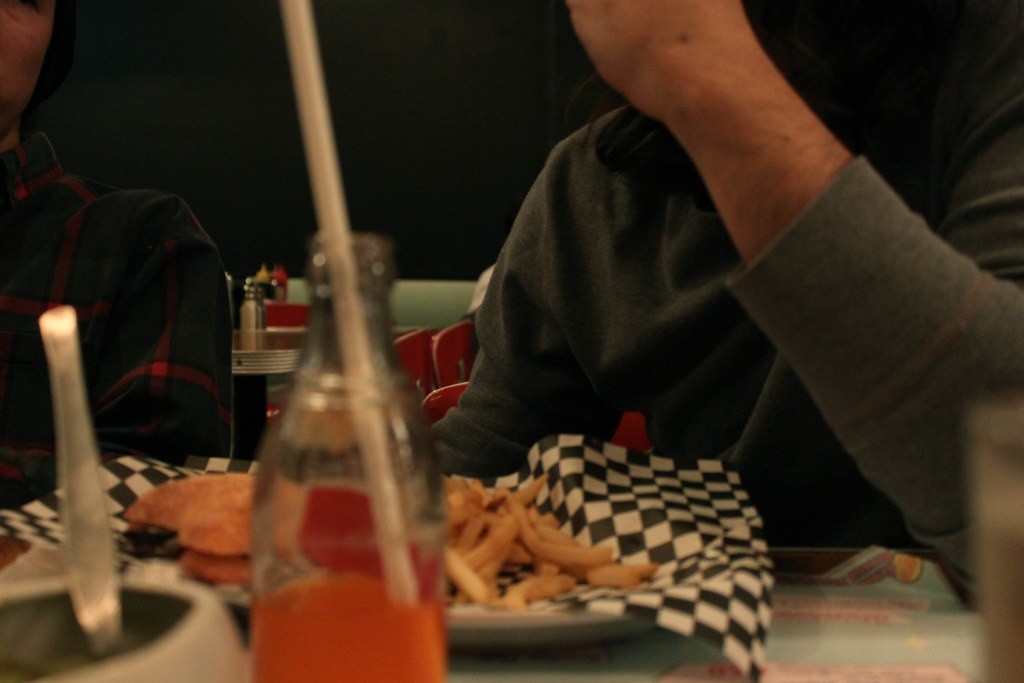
left=123, top=473, right=305, bottom=586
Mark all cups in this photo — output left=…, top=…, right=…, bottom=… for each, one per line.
left=0, top=574, right=247, bottom=683
left=971, top=404, right=1024, bottom=683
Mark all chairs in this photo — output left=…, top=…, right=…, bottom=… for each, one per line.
left=262, top=298, right=479, bottom=422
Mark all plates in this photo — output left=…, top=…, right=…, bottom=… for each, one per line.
left=171, top=562, right=660, bottom=647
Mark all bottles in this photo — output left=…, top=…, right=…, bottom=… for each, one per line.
left=255, top=266, right=286, bottom=300
left=248, top=232, right=447, bottom=682
left=240, top=286, right=267, bottom=330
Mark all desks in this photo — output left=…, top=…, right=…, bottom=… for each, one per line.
left=0, top=441, right=987, bottom=683
left=228, top=329, right=310, bottom=454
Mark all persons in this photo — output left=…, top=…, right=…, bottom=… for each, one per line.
left=432, top=0, right=1024, bottom=610
left=0, top=0, right=233, bottom=510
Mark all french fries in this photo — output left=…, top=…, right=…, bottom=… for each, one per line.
left=437, top=475, right=658, bottom=607
